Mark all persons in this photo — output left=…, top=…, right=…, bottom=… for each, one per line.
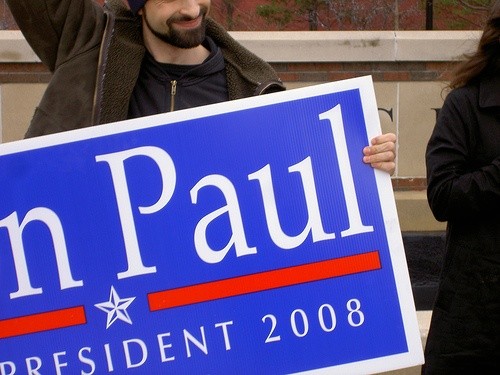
left=6, top=0, right=397, bottom=180
left=420, top=0, right=499, bottom=375
